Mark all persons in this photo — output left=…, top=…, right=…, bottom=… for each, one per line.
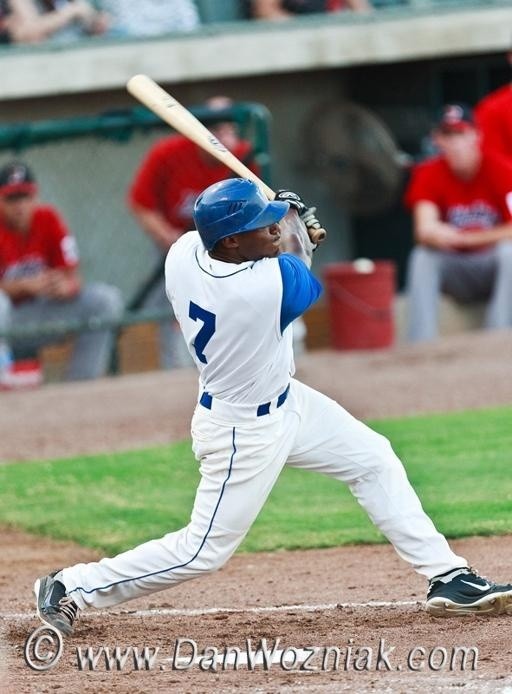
left=129, top=95, right=258, bottom=373
left=400, top=101, right=512, bottom=345
left=0, top=1, right=202, bottom=43
left=0, top=159, right=126, bottom=387
left=32, top=177, right=512, bottom=636
left=252, top=0, right=374, bottom=20
left=473, top=82, right=511, bottom=162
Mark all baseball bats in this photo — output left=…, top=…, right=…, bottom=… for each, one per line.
left=127, top=74, right=326, bottom=244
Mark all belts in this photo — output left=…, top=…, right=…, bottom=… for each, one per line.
left=200, top=384, right=290, bottom=417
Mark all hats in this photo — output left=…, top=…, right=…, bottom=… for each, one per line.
left=1, top=161, right=40, bottom=197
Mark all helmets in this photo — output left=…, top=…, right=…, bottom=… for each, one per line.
left=191, top=176, right=290, bottom=250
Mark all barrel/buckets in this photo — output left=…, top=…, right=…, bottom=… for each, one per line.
left=321, top=261, right=395, bottom=351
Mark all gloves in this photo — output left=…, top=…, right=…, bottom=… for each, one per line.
left=270, top=189, right=328, bottom=254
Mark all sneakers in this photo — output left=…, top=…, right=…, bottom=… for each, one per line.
left=422, top=566, right=512, bottom=622
left=33, top=568, right=80, bottom=638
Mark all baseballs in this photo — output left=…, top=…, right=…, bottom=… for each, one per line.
left=353, top=257, right=375, bottom=275
